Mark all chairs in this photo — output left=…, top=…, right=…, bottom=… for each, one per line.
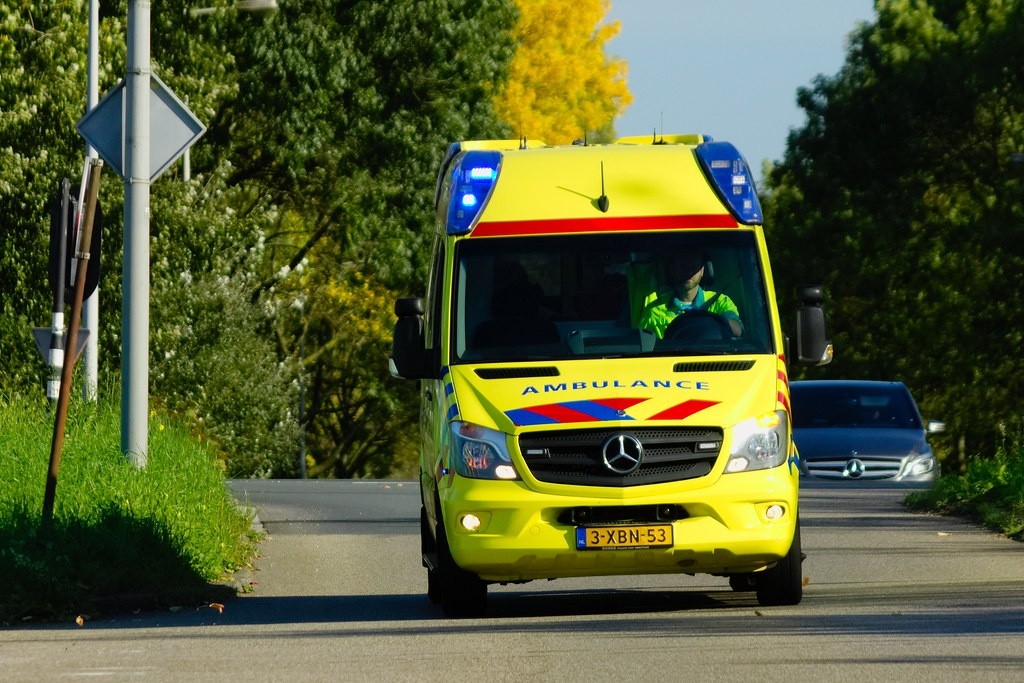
left=470, top=286, right=561, bottom=350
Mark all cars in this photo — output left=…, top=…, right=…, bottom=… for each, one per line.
left=786, top=380, right=948, bottom=490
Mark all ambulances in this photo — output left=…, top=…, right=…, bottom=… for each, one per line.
left=386, top=133, right=828, bottom=608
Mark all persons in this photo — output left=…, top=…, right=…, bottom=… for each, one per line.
left=639, top=253, right=742, bottom=340
